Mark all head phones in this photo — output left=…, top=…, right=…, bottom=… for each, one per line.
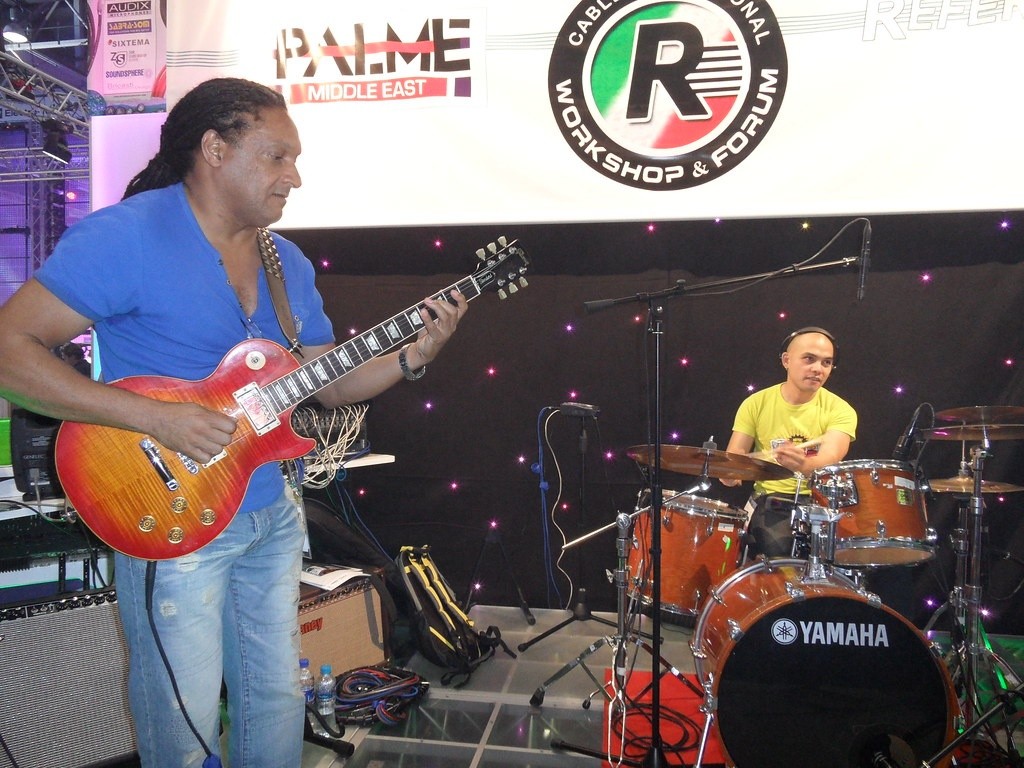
left=777, top=327, right=840, bottom=378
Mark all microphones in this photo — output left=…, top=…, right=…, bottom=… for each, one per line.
left=560, top=401, right=599, bottom=417
left=892, top=406, right=921, bottom=461
left=856, top=221, right=872, bottom=301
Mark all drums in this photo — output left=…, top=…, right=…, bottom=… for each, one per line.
left=806, top=459, right=938, bottom=566
left=627, top=488, right=749, bottom=617
left=689, top=556, right=961, bottom=768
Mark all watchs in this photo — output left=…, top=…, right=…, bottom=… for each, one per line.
left=399, top=343, right=426, bottom=382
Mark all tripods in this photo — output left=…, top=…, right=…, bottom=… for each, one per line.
left=529, top=456, right=707, bottom=710
left=514, top=416, right=665, bottom=652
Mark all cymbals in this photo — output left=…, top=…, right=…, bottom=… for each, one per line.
left=623, top=444, right=795, bottom=481
left=920, top=424, right=1024, bottom=440
left=935, top=405, right=1024, bottom=423
left=927, top=476, right=1024, bottom=493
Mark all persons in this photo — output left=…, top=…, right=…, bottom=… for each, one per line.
left=0, top=79, right=469, bottom=767
left=718, top=327, right=858, bottom=562
left=60, top=342, right=92, bottom=377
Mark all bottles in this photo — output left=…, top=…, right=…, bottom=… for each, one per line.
left=317, top=664, right=336, bottom=737
left=300, top=658, right=316, bottom=734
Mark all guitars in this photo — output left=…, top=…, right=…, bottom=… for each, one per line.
left=54, top=236, right=533, bottom=561
